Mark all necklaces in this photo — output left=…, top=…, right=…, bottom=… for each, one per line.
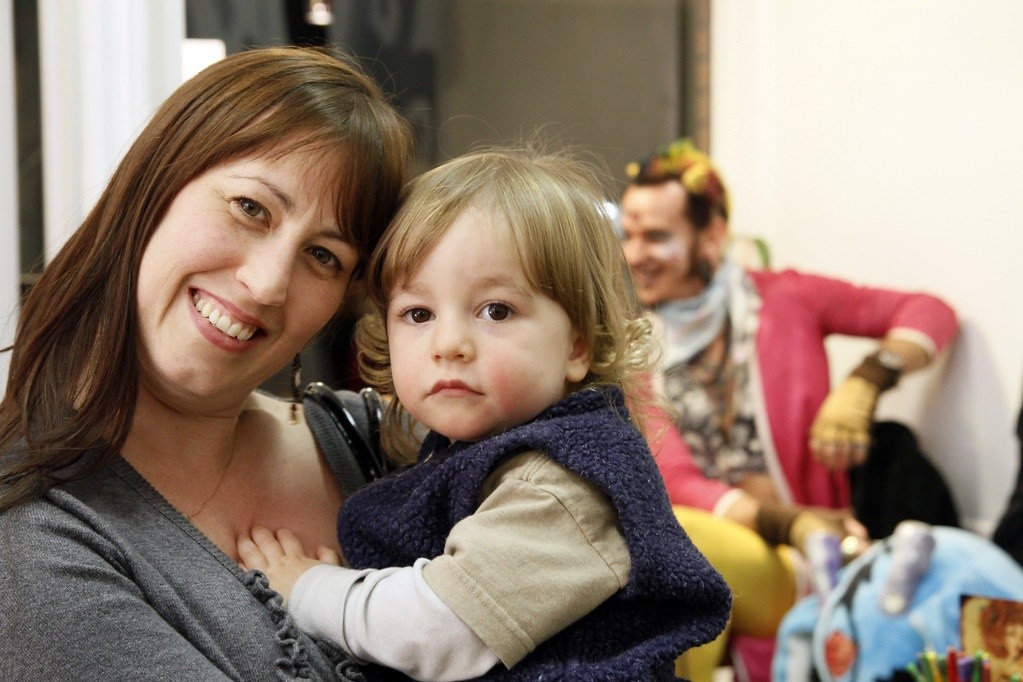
left=180, top=432, right=236, bottom=522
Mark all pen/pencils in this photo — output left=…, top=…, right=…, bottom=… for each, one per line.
left=904, top=646, right=1022, bottom=682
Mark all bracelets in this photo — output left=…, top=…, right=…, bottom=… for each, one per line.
left=850, top=356, right=899, bottom=391
left=878, top=352, right=905, bottom=371
left=755, top=498, right=807, bottom=549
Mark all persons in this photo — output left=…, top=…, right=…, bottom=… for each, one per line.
left=1, top=45, right=429, bottom=682
left=236, top=149, right=733, bottom=681
left=622, top=137, right=962, bottom=566
